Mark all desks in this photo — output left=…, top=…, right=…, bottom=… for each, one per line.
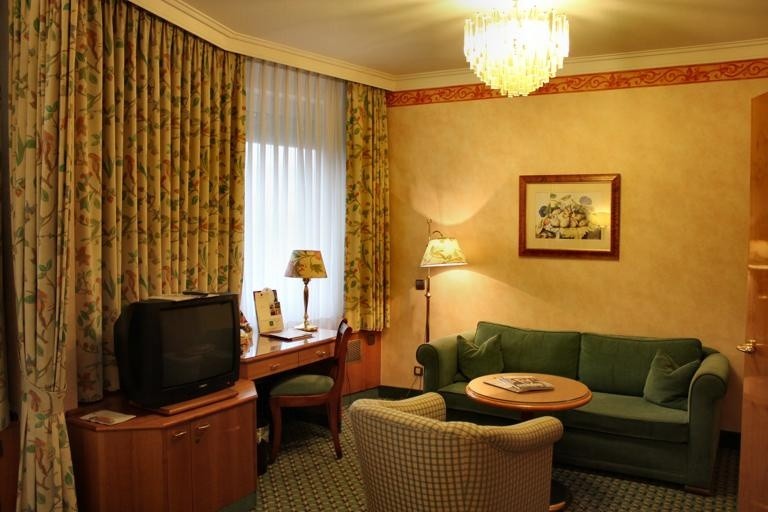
left=239, top=328, right=338, bottom=382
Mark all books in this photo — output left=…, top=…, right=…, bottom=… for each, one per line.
left=485, top=374, right=554, bottom=395
left=81, top=408, right=136, bottom=426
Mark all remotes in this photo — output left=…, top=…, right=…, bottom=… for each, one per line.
left=182, top=291, right=208, bottom=295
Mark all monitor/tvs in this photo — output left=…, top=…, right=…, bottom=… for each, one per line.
left=113, top=294, right=240, bottom=409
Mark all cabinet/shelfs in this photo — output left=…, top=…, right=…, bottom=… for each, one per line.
left=66, top=379, right=258, bottom=511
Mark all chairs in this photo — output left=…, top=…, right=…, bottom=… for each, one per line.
left=268, top=317, right=353, bottom=464
left=347, top=391, right=563, bottom=511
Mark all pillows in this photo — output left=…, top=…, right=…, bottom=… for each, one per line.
left=641, top=347, right=701, bottom=411
left=454, top=333, right=507, bottom=381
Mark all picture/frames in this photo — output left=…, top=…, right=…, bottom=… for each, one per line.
left=518, top=174, right=621, bottom=260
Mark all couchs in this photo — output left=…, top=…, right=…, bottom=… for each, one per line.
left=414, top=320, right=729, bottom=495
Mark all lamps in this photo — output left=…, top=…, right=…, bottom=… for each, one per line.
left=462, top=3, right=570, bottom=96
left=419, top=218, right=468, bottom=344
left=283, top=250, right=328, bottom=330
